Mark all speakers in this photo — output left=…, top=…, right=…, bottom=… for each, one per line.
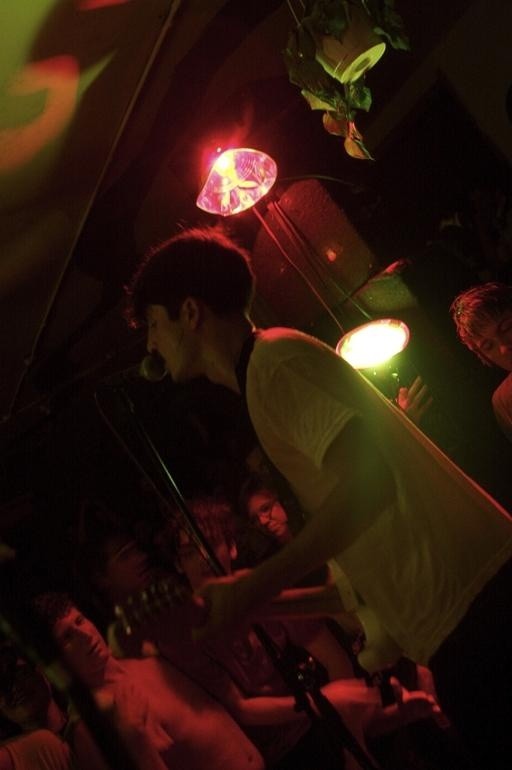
left=249, top=155, right=442, bottom=329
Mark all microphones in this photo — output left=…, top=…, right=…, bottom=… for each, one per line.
left=94, top=355, right=168, bottom=388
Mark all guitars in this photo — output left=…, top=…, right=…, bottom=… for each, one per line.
left=106, top=567, right=404, bottom=674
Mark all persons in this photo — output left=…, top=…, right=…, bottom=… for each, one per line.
left=122, top=222, right=511, bottom=769
